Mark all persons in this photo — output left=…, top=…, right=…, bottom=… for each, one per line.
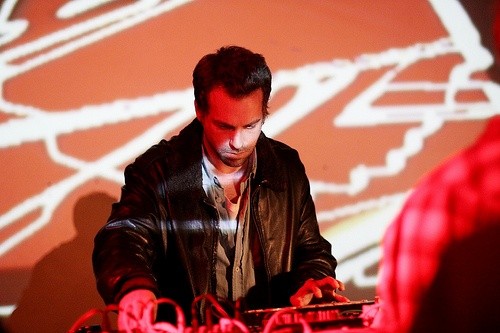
left=89, top=44, right=354, bottom=331
